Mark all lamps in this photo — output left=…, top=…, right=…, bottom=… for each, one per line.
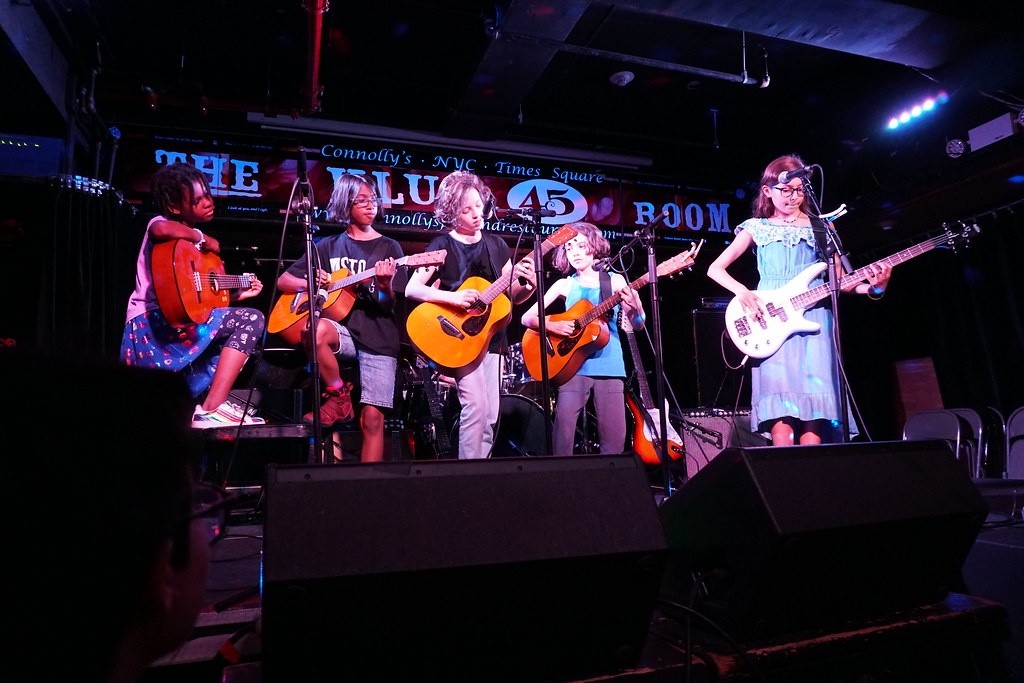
left=945, top=109, right=1024, bottom=160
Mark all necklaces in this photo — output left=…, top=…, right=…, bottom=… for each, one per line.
left=774, top=210, right=801, bottom=223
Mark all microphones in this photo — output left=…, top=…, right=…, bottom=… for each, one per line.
left=778, top=165, right=813, bottom=184
left=715, top=433, right=723, bottom=448
left=314, top=289, right=329, bottom=321
left=591, top=257, right=611, bottom=271
left=480, top=195, right=494, bottom=219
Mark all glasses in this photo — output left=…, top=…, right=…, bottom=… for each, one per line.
left=769, top=185, right=807, bottom=197
left=349, top=193, right=383, bottom=208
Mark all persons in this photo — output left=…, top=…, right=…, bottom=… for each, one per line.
left=278, top=171, right=646, bottom=466
left=120, top=161, right=267, bottom=431
left=707, top=157, right=893, bottom=447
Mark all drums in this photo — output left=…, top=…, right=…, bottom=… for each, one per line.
left=505, top=342, right=537, bottom=386
left=450, top=378, right=560, bottom=456
left=406, top=381, right=451, bottom=424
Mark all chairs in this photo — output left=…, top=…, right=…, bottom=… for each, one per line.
left=902, top=405, right=1024, bottom=480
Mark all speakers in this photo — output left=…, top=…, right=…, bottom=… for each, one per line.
left=690, top=309, right=754, bottom=415
left=262, top=407, right=987, bottom=660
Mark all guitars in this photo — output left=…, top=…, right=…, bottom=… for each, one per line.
left=404, top=223, right=578, bottom=380
left=149, top=237, right=259, bottom=329
left=726, top=217, right=982, bottom=362
left=521, top=236, right=705, bottom=386
left=416, top=353, right=452, bottom=460
left=618, top=305, right=685, bottom=466
left=266, top=247, right=448, bottom=348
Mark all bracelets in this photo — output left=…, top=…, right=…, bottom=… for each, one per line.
left=634, top=312, right=646, bottom=323
left=873, top=288, right=886, bottom=294
left=192, top=228, right=205, bottom=250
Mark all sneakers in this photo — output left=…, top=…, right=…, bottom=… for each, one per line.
left=303, top=382, right=355, bottom=428
left=191, top=401, right=266, bottom=429
